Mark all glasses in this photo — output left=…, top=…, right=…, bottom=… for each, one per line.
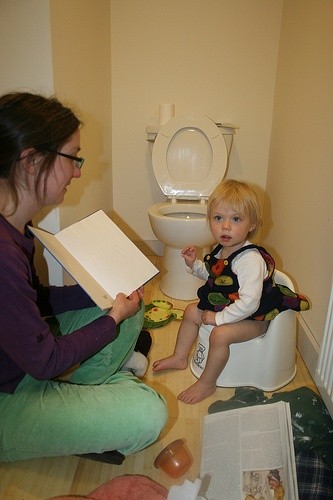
left=34, top=147, right=85, bottom=169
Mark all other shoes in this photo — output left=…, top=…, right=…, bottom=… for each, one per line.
left=74, top=449, right=126, bottom=466
left=121, top=330, right=153, bottom=376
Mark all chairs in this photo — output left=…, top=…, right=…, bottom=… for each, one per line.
left=188, top=268, right=299, bottom=393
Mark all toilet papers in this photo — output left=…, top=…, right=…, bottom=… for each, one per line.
left=159, top=103, right=175, bottom=126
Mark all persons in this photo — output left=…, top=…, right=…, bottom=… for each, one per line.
left=152, top=179, right=310, bottom=404
left=0, top=90, right=170, bottom=464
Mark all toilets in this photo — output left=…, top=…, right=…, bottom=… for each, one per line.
left=147, top=110, right=236, bottom=301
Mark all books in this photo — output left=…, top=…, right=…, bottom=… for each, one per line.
left=202, top=401, right=300, bottom=500
left=25, top=207, right=161, bottom=313
